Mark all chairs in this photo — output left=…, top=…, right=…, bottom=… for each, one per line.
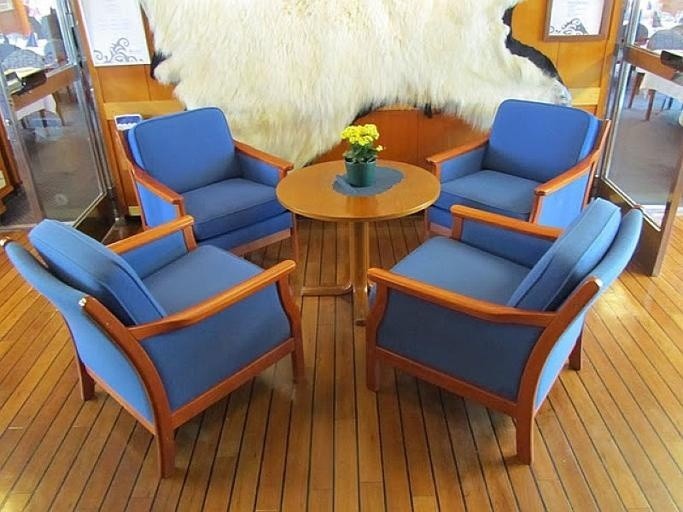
left=366, top=197, right=644, bottom=466
left=423, top=98, right=612, bottom=239
left=619, top=23, right=683, bottom=50
left=1, top=214, right=305, bottom=480
left=113, top=107, right=302, bottom=271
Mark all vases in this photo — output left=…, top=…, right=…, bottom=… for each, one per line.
left=346, top=162, right=376, bottom=188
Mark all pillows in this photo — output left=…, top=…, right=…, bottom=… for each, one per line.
left=484, top=99, right=598, bottom=184
left=26, top=219, right=167, bottom=327
left=129, top=107, right=241, bottom=197
left=503, top=197, right=621, bottom=314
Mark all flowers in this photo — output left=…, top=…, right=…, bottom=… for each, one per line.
left=342, top=122, right=384, bottom=163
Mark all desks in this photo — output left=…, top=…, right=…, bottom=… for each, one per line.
left=627, top=49, right=683, bottom=109
left=3, top=49, right=80, bottom=127
left=641, top=71, right=683, bottom=126
